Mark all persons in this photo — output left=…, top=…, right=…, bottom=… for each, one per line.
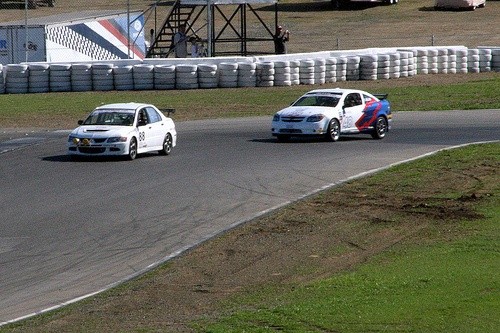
left=149, top=28, right=156, bottom=59
left=175, top=24, right=187, bottom=59
left=188, top=37, right=200, bottom=59
left=274, top=26, right=290, bottom=54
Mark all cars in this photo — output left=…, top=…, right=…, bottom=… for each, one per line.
left=65, top=101, right=178, bottom=160
left=270, top=87, right=394, bottom=142
